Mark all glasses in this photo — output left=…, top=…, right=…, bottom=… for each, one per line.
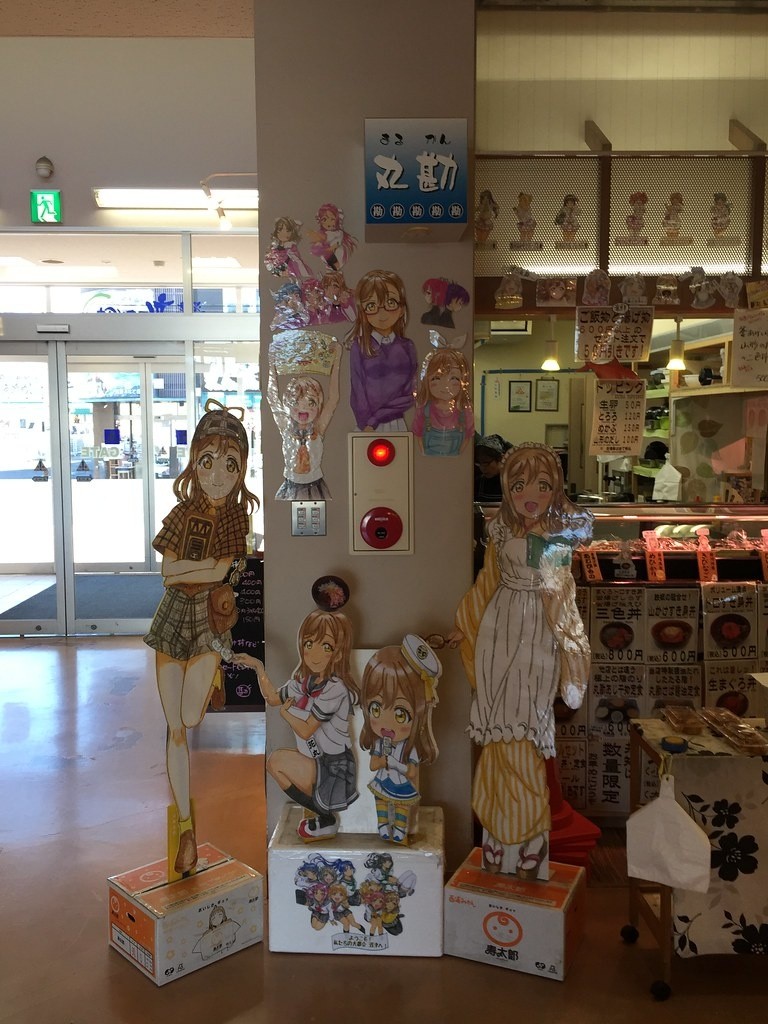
left=475, top=457, right=497, bottom=467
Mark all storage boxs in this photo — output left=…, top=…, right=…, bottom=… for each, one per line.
left=267, top=800, right=444, bottom=957
left=107, top=841, right=264, bottom=988
left=443, top=846, right=588, bottom=983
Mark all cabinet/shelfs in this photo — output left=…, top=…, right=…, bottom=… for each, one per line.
left=600, top=326, right=768, bottom=506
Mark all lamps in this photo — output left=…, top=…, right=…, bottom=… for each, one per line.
left=541, top=320, right=562, bottom=372
left=665, top=317, right=687, bottom=371
left=91, top=186, right=260, bottom=230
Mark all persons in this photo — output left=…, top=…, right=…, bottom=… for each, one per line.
left=474, top=434, right=511, bottom=500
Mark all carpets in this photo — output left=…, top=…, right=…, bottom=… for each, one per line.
left=0, top=573, right=165, bottom=622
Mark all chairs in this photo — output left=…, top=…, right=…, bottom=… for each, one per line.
left=109, top=460, right=119, bottom=479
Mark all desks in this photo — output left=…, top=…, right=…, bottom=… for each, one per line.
left=115, top=466, right=135, bottom=479
left=619, top=719, right=768, bottom=998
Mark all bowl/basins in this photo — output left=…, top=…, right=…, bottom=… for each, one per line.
left=553, top=697, right=578, bottom=720
left=716, top=691, right=748, bottom=716
left=710, top=614, right=751, bottom=649
left=598, top=622, right=635, bottom=649
left=611, top=711, right=623, bottom=722
left=626, top=706, right=639, bottom=718
left=662, top=736, right=689, bottom=752
left=651, top=620, right=691, bottom=644
left=595, top=706, right=608, bottom=719
left=682, top=375, right=712, bottom=386
